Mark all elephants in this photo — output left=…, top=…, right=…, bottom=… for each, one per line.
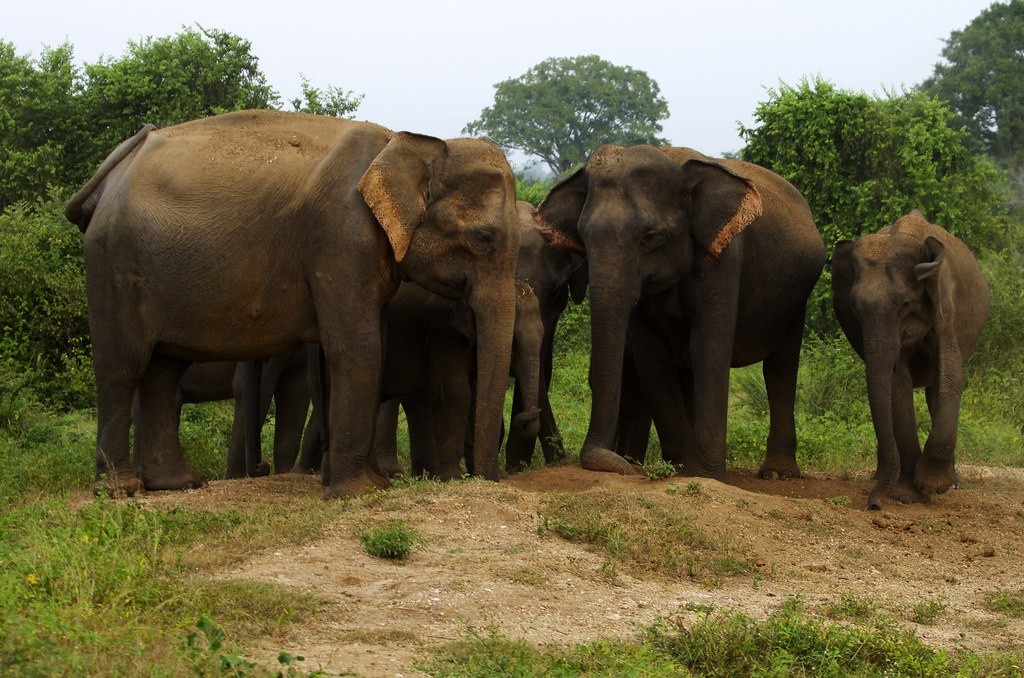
left=61, top=108, right=991, bottom=510
